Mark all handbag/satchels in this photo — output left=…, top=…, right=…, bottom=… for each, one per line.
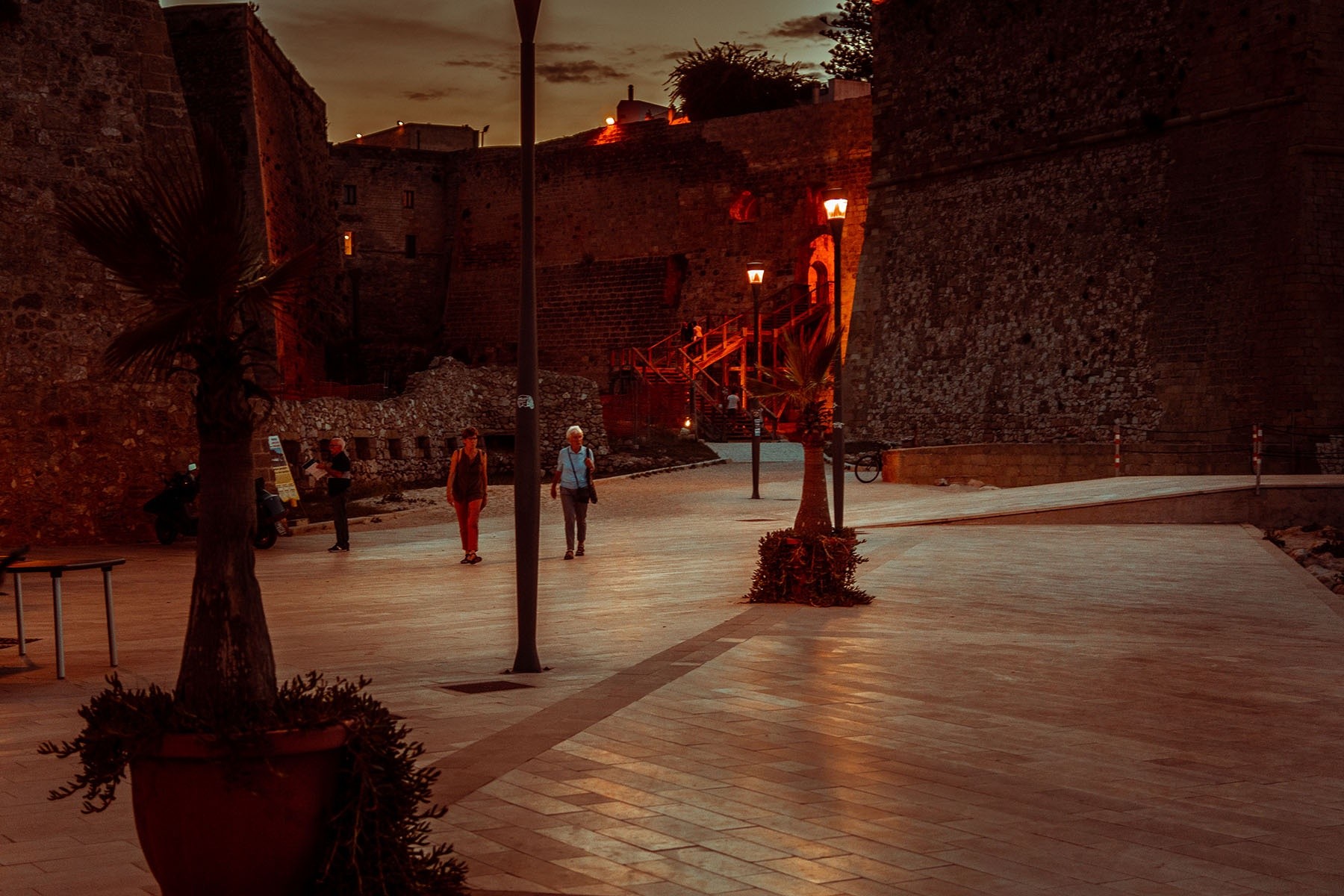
left=573, top=486, right=589, bottom=504
left=589, top=483, right=599, bottom=504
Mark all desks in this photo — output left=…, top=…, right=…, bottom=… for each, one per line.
left=5, top=558, right=128, bottom=678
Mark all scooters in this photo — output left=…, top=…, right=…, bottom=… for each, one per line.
left=144, top=463, right=281, bottom=549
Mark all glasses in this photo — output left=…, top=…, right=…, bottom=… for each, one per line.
left=329, top=444, right=341, bottom=447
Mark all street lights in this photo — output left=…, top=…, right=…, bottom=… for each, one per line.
left=820, top=187, right=850, bottom=534
left=745, top=261, right=766, bottom=501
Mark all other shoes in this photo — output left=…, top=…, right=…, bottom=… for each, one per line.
left=565, top=550, right=574, bottom=560
left=576, top=544, right=585, bottom=556
left=328, top=544, right=348, bottom=553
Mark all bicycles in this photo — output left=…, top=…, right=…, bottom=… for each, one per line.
left=854, top=440, right=883, bottom=483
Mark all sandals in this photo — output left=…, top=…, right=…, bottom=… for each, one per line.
left=461, top=553, right=470, bottom=563
left=469, top=553, right=482, bottom=564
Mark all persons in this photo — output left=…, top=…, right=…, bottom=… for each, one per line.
left=446, top=426, right=488, bottom=565
left=726, top=390, right=741, bottom=422
left=309, top=438, right=352, bottom=552
left=682, top=320, right=703, bottom=356
left=550, top=425, right=595, bottom=560
